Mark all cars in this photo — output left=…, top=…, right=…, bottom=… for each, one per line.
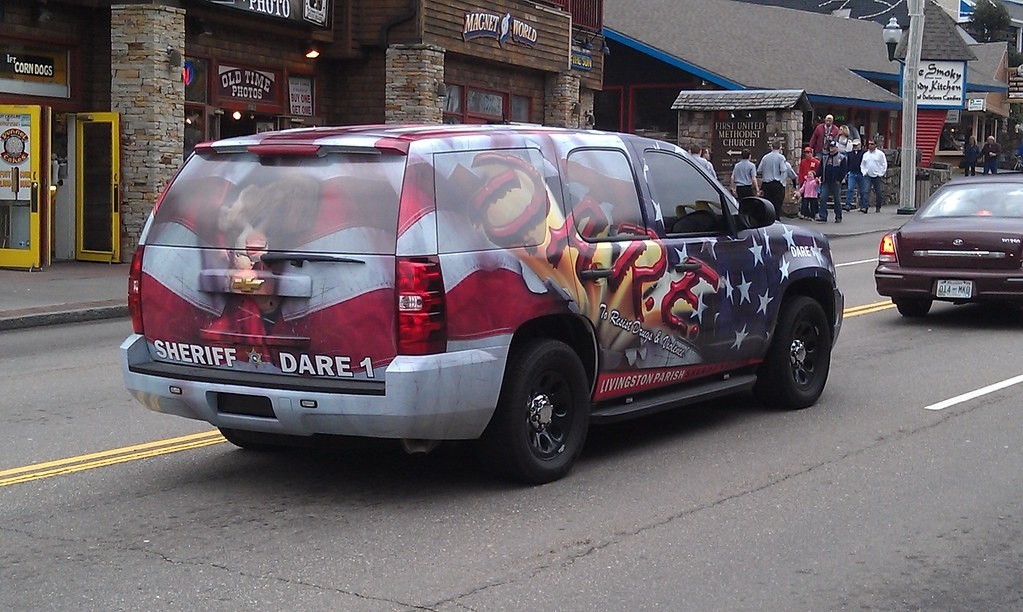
left=875, top=174, right=1023, bottom=317
left=118, top=121, right=842, bottom=482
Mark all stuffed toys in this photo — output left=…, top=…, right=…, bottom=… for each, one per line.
left=792, top=191, right=802, bottom=204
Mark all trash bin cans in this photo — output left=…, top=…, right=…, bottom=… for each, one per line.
left=915, top=175, right=930, bottom=210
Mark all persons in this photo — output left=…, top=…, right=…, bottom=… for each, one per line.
left=757, top=141, right=799, bottom=222
left=859, top=140, right=887, bottom=213
left=961, top=136, right=1002, bottom=177
left=731, top=148, right=759, bottom=199
left=685, top=144, right=718, bottom=179
left=798, top=115, right=864, bottom=223
left=944, top=126, right=959, bottom=147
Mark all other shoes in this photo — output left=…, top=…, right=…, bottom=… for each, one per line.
left=797, top=212, right=819, bottom=221
left=858, top=207, right=868, bottom=213
left=815, top=217, right=827, bottom=223
left=875, top=207, right=880, bottom=212
left=835, top=218, right=842, bottom=223
left=845, top=206, right=850, bottom=211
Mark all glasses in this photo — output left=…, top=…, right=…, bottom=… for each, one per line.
left=867, top=143, right=875, bottom=145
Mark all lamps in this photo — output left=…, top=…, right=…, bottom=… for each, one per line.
left=728, top=111, right=736, bottom=120
left=167, top=46, right=182, bottom=68
left=746, top=111, right=753, bottom=118
left=434, top=81, right=447, bottom=100
left=590, top=32, right=610, bottom=54
left=571, top=28, right=593, bottom=50
left=571, top=102, right=580, bottom=115
left=585, top=110, right=595, bottom=125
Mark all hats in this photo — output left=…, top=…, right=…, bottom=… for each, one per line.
left=852, top=139, right=860, bottom=145
left=804, top=147, right=812, bottom=153
left=825, top=115, right=833, bottom=121
left=829, top=141, right=838, bottom=148
